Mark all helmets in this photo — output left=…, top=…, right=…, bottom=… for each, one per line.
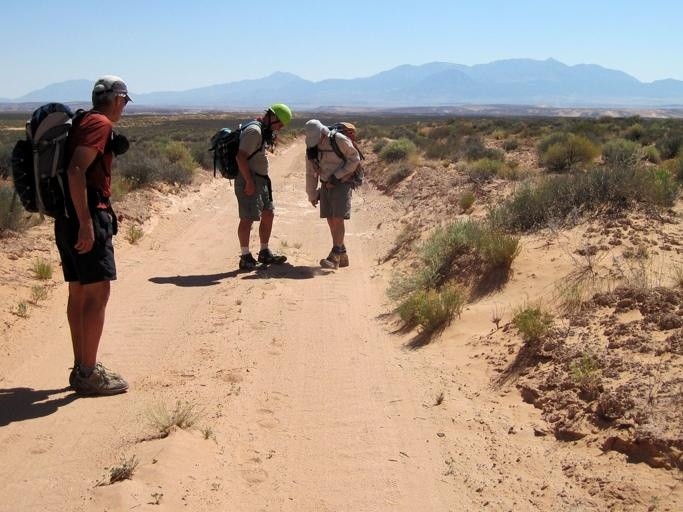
left=269, top=104, right=292, bottom=126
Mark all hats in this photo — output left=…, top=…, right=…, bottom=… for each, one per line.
left=305, top=119, right=321, bottom=144
left=93, top=77, right=133, bottom=104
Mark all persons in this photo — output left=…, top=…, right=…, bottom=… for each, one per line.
left=302, top=119, right=361, bottom=269
left=54, top=75, right=134, bottom=393
left=233, top=104, right=292, bottom=270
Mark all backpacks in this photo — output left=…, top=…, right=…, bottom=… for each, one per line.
left=11, top=104, right=85, bottom=219
left=328, top=122, right=365, bottom=184
left=209, top=121, right=264, bottom=179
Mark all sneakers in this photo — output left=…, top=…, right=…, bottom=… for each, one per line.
left=320, top=252, right=348, bottom=269
left=69, top=362, right=128, bottom=395
left=240, top=250, right=287, bottom=270
